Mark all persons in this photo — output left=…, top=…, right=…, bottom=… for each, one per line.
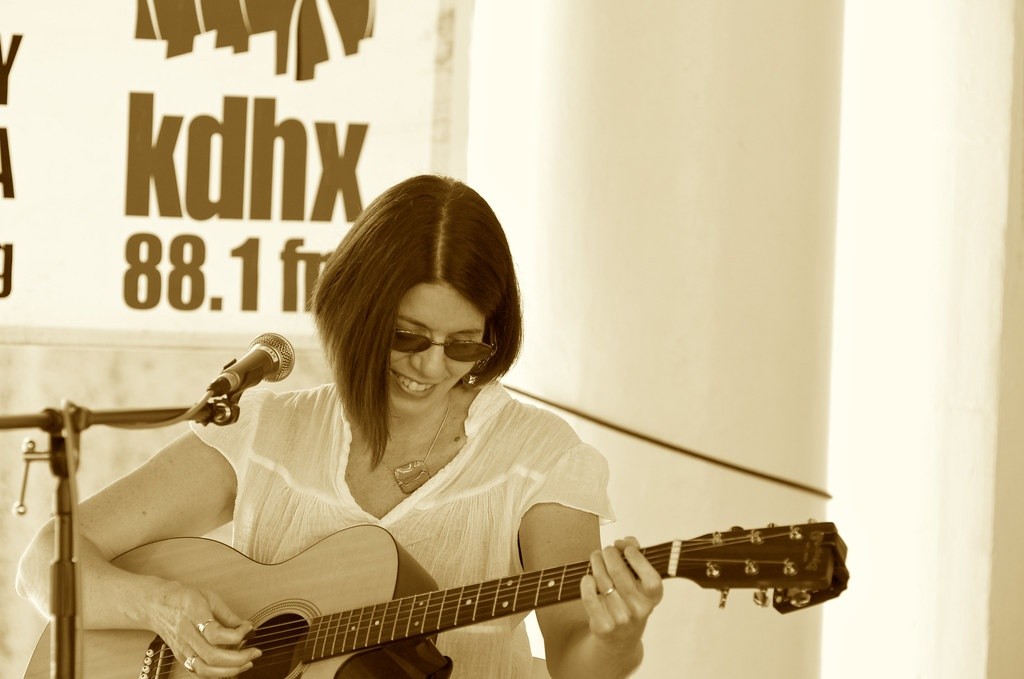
left=16, top=175, right=664, bottom=679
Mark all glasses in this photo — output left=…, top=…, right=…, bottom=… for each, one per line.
left=392, top=321, right=497, bottom=362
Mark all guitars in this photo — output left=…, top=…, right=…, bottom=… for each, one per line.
left=22, top=514, right=851, bottom=679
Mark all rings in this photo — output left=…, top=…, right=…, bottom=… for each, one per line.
left=197, top=618, right=216, bottom=635
left=183, top=653, right=198, bottom=672
left=603, top=586, right=616, bottom=596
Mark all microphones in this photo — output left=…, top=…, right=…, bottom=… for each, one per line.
left=206, top=332, right=295, bottom=396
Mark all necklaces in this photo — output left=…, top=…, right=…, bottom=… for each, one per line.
left=372, top=385, right=448, bottom=495
